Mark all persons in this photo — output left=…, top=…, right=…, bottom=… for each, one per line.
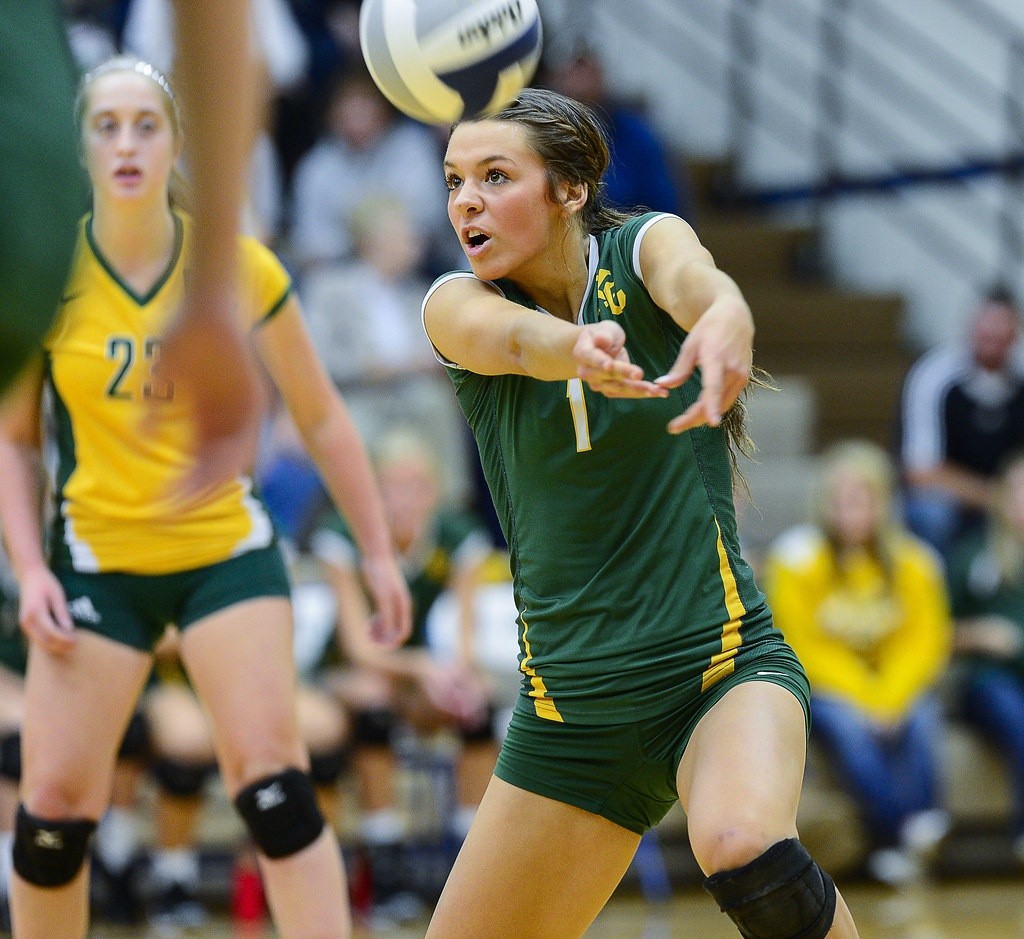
left=0, top=1, right=268, bottom=512
left=0, top=0, right=682, bottom=939
left=0, top=54, right=414, bottom=939
left=420, top=86, right=861, bottom=938
left=762, top=278, right=1024, bottom=887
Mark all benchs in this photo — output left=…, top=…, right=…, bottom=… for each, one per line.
left=136, top=95, right=1024, bottom=847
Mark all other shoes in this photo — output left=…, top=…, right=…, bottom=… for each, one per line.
left=869, top=815, right=972, bottom=886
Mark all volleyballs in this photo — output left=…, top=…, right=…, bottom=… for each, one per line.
left=359, top=2, right=545, bottom=127
